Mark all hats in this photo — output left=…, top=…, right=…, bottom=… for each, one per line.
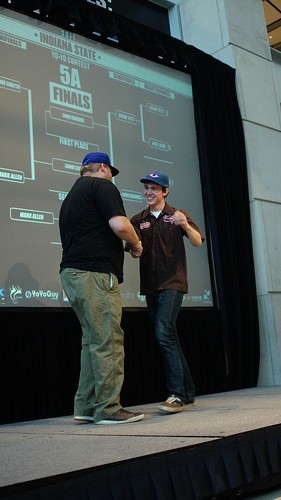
left=140, top=172, right=169, bottom=188
left=81, top=152, right=119, bottom=177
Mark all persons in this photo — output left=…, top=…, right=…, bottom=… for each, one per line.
left=59, top=151, right=144, bottom=425
left=126, top=173, right=205, bottom=413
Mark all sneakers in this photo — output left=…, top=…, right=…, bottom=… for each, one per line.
left=157, top=395, right=185, bottom=412
left=95, top=409, right=145, bottom=424
